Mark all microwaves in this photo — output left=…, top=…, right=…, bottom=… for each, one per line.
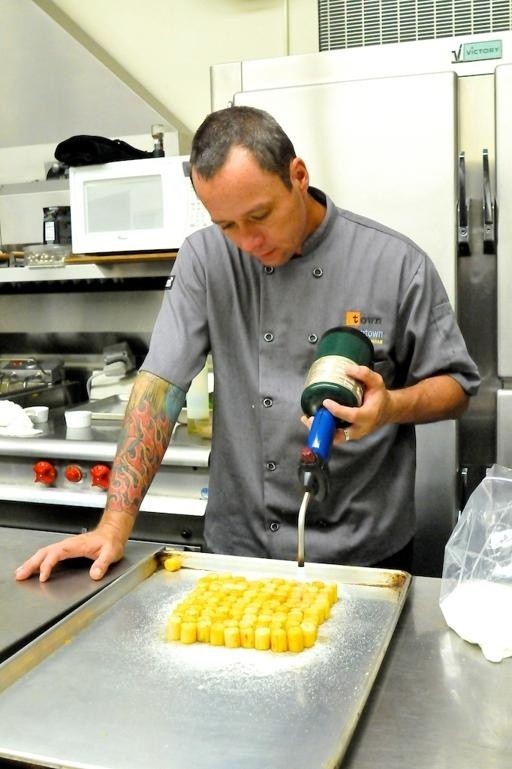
left=69, top=154, right=215, bottom=257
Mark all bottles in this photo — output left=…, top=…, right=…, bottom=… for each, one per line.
left=299, top=326, right=375, bottom=493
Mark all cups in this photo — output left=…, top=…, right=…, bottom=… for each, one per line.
left=24, top=405, right=49, bottom=425
left=63, top=410, right=93, bottom=428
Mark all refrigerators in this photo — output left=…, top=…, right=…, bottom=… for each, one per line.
left=206, top=29, right=512, bottom=586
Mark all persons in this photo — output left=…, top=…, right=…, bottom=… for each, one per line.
left=14, top=103, right=482, bottom=583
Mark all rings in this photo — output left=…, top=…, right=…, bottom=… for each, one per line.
left=345, top=430, right=350, bottom=441
